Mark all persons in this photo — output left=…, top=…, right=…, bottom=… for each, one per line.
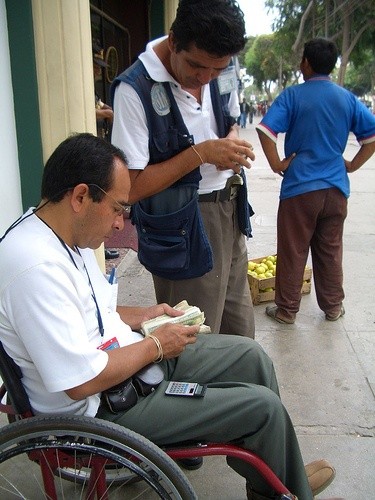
left=93, top=42, right=113, bottom=120
left=236, top=98, right=272, bottom=128
left=255, top=38, right=375, bottom=323
left=0, top=135, right=335, bottom=500
left=111, top=1, right=255, bottom=470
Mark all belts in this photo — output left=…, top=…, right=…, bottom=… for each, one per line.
left=199, top=184, right=238, bottom=202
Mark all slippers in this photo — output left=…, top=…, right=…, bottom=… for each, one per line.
left=265, top=305, right=288, bottom=324
left=326, top=305, right=345, bottom=321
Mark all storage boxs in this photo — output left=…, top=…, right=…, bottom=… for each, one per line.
left=246, top=254, right=312, bottom=305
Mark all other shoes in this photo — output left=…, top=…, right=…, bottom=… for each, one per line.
left=246, top=458, right=346, bottom=500
left=177, top=455, right=203, bottom=470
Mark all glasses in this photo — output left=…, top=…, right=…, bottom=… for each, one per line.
left=67, top=183, right=130, bottom=217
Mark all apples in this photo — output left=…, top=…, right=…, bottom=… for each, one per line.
left=247, top=255, right=277, bottom=292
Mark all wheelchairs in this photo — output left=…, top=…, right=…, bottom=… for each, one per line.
left=0, top=330, right=300, bottom=500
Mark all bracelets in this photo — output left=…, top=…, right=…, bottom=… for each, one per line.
left=190, top=145, right=204, bottom=165
left=147, top=335, right=163, bottom=364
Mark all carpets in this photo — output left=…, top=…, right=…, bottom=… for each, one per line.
left=104, top=213, right=138, bottom=252
